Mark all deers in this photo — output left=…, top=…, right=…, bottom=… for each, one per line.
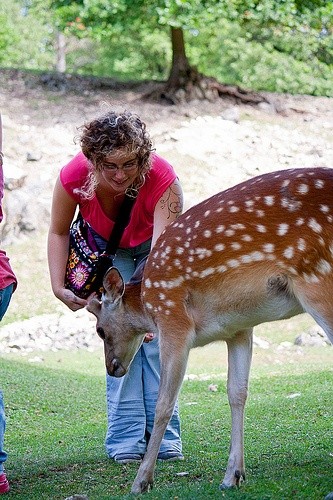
left=85, top=165, right=333, bottom=496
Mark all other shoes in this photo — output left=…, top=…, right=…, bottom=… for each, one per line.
left=0, top=473, right=9, bottom=494
left=158, top=449, right=184, bottom=462
left=115, top=454, right=142, bottom=464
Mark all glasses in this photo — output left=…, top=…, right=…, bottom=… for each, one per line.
left=100, top=160, right=139, bottom=173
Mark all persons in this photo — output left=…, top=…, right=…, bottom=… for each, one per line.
left=0, top=113, right=18, bottom=495
left=48, top=110, right=184, bottom=464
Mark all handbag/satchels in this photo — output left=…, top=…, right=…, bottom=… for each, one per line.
left=64, top=221, right=116, bottom=299
